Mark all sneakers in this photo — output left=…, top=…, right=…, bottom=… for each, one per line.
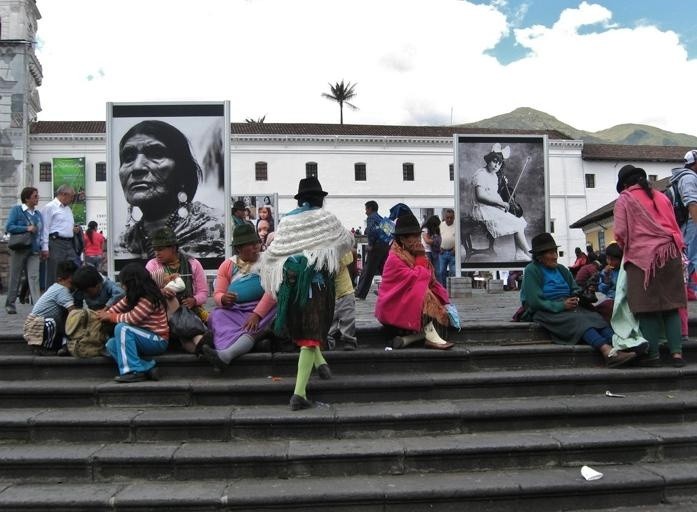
left=606, top=351, right=663, bottom=368
left=113, top=358, right=161, bottom=382
left=6, top=305, right=17, bottom=314
left=671, top=358, right=686, bottom=367
left=193, top=331, right=229, bottom=371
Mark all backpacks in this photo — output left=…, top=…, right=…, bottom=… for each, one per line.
left=661, top=178, right=689, bottom=226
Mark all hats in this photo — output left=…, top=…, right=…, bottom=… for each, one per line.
left=231, top=201, right=246, bottom=211
left=229, top=224, right=262, bottom=246
left=294, top=177, right=328, bottom=199
left=683, top=150, right=697, bottom=165
left=390, top=215, right=424, bottom=235
left=617, top=165, right=646, bottom=193
left=149, top=227, right=182, bottom=247
left=528, top=232, right=560, bottom=253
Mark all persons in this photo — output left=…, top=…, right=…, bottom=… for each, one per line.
left=258, top=177, right=355, bottom=411
left=0, top=175, right=458, bottom=384
left=117, top=119, right=223, bottom=253
left=517, top=146, right=697, bottom=368
left=467, top=151, right=534, bottom=259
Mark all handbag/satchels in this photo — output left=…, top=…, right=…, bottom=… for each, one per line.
left=65, top=307, right=110, bottom=358
left=228, top=273, right=265, bottom=304
left=8, top=232, right=33, bottom=251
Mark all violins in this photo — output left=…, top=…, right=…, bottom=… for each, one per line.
left=498, top=167, right=524, bottom=216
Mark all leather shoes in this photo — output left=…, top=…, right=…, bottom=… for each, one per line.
left=318, top=364, right=333, bottom=380
left=393, top=336, right=404, bottom=349
left=290, top=394, right=313, bottom=411
left=424, top=339, right=455, bottom=350
